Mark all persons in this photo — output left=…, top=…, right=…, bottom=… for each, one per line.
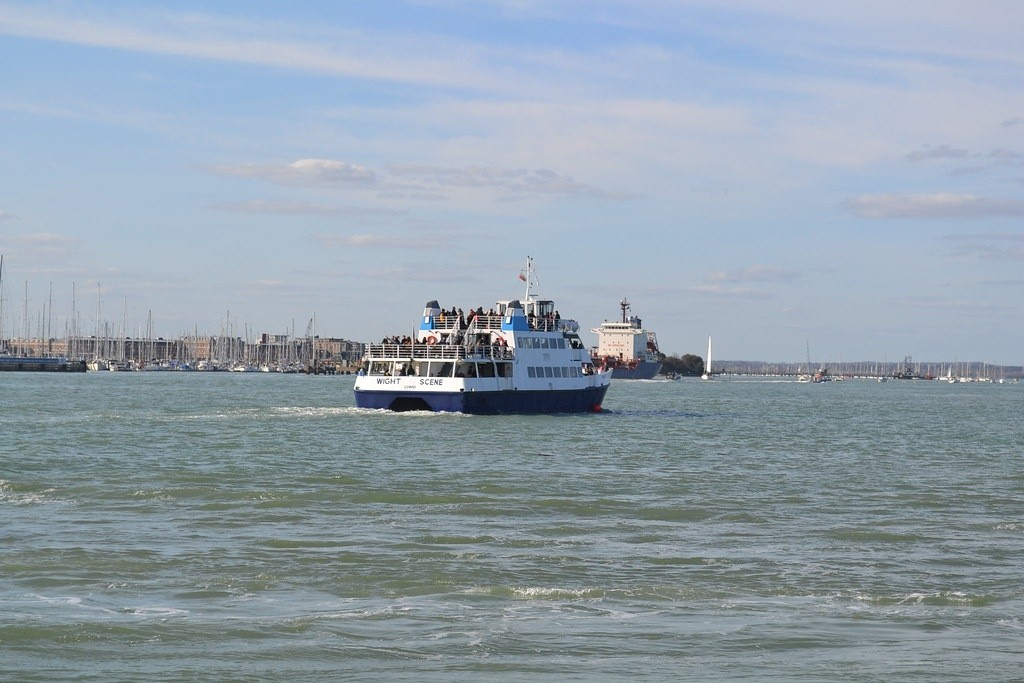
left=359, top=307, right=608, bottom=376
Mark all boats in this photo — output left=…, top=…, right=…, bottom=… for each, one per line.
left=352, top=253, right=614, bottom=416
left=586, top=297, right=667, bottom=380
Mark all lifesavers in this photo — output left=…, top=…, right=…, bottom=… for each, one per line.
left=426, top=335, right=436, bottom=344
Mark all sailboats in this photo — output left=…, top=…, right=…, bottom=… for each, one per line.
left=700, top=335, right=712, bottom=380
left=83, top=312, right=353, bottom=374
